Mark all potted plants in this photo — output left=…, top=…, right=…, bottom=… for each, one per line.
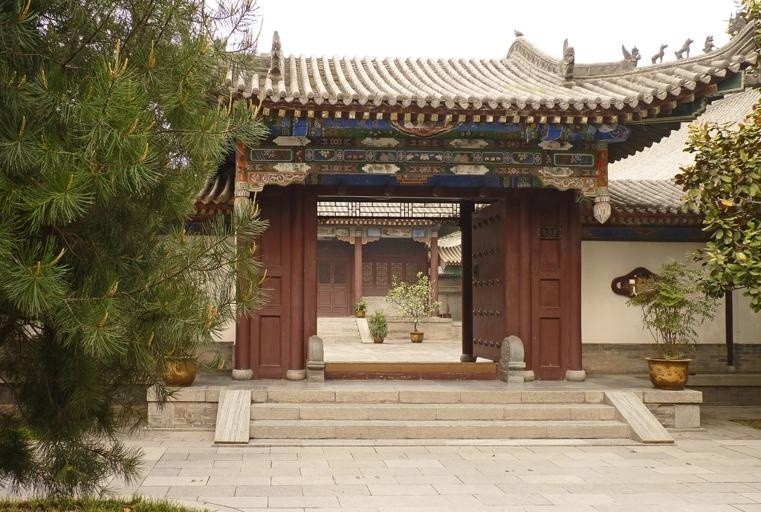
left=146, top=278, right=226, bottom=387
left=352, top=295, right=368, bottom=318
left=365, top=308, right=390, bottom=343
left=383, top=270, right=444, bottom=343
left=622, top=248, right=723, bottom=391
